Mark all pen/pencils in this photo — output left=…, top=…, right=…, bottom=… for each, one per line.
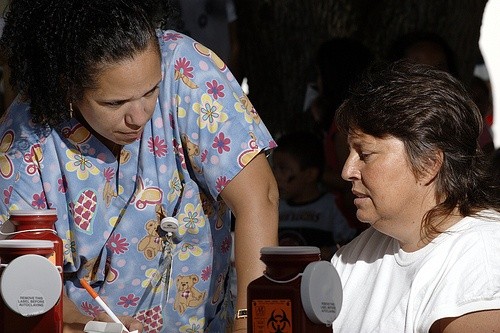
left=79, top=278, right=132, bottom=333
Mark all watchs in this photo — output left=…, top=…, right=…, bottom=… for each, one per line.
left=233, top=308, right=248, bottom=319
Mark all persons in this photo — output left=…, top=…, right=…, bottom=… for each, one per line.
left=0, top=0, right=283, bottom=333
left=325, top=63, right=500, bottom=332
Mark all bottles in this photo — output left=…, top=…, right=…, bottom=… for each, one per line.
left=247, top=247, right=343, bottom=333
left=0, top=209, right=64, bottom=333
